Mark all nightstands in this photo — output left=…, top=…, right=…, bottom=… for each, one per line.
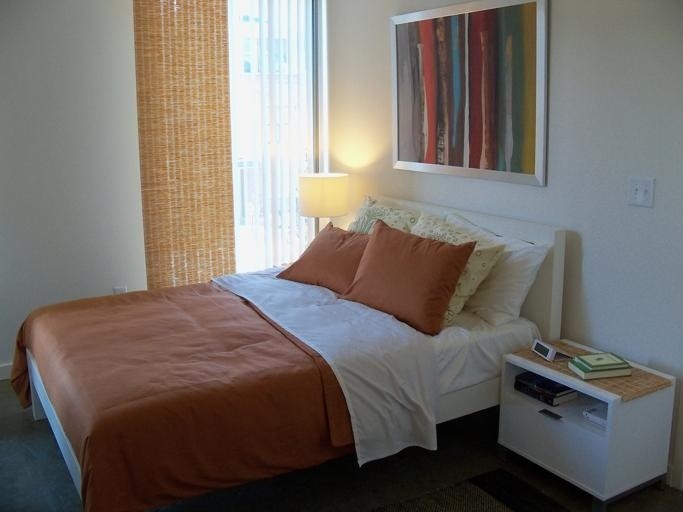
left=498, top=339, right=677, bottom=501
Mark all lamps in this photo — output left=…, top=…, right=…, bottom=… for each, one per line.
left=296, top=173, right=351, bottom=227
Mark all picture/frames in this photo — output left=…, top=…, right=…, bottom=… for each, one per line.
left=391, top=0, right=547, bottom=189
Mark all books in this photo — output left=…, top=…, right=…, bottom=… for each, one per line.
left=567, top=360, right=634, bottom=382
left=514, top=370, right=577, bottom=397
left=574, top=350, right=629, bottom=369
left=513, top=381, right=579, bottom=406
left=582, top=401, right=609, bottom=428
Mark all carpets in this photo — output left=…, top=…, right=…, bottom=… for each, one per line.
left=368, top=467, right=573, bottom=512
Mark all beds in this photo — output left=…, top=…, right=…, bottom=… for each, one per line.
left=11, top=195, right=565, bottom=511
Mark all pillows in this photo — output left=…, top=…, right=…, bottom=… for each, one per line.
left=444, top=213, right=549, bottom=327
left=408, top=212, right=509, bottom=327
left=335, top=220, right=478, bottom=336
left=346, top=195, right=421, bottom=236
left=275, top=220, right=372, bottom=294
